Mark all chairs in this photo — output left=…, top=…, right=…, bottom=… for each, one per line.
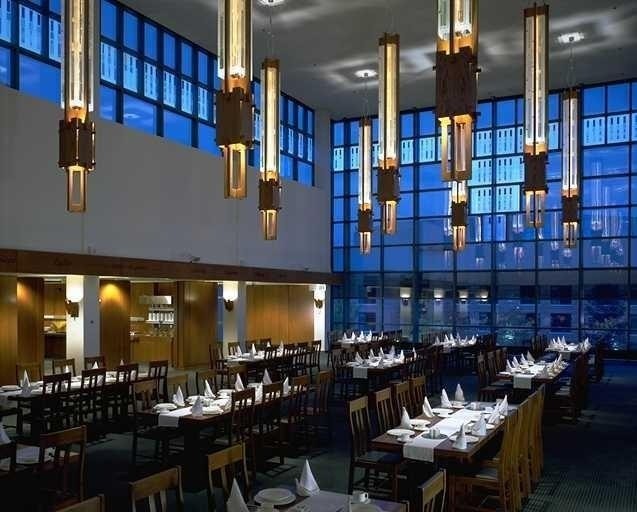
left=0, top=329, right=607, bottom=510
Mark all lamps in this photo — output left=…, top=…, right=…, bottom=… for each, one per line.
left=313, top=289, right=325, bottom=309
left=523, top=1, right=550, bottom=229
left=58, top=0, right=100, bottom=212
left=352, top=68, right=377, bottom=255
left=433, top=1, right=482, bottom=253
left=378, top=0, right=400, bottom=233
left=64, top=274, right=83, bottom=317
left=216, top=0, right=257, bottom=199
left=558, top=31, right=584, bottom=248
left=259, top=0, right=281, bottom=241
left=222, top=281, right=238, bottom=310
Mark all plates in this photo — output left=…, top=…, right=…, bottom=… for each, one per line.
left=255, top=488, right=297, bottom=506
left=409, top=419, right=432, bottom=426
left=468, top=423, right=495, bottom=430
left=449, top=435, right=479, bottom=443
left=387, top=428, right=416, bottom=437
left=480, top=414, right=506, bottom=420
left=432, top=408, right=455, bottom=414
left=258, top=488, right=292, bottom=501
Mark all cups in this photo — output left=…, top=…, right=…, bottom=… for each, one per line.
left=353, top=490, right=369, bottom=503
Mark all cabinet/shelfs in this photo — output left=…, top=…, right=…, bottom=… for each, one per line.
left=145, top=308, right=175, bottom=325
